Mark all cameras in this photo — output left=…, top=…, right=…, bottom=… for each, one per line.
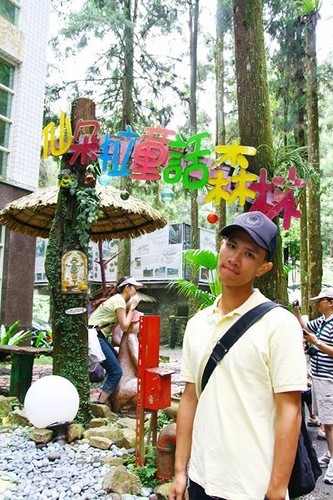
left=293, top=300, right=299, bottom=309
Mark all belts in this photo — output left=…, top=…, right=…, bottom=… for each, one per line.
left=88, top=325, right=98, bottom=328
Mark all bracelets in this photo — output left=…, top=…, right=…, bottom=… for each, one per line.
left=315, top=340, right=321, bottom=347
left=130, top=308, right=134, bottom=311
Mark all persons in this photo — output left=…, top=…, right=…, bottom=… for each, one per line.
left=88, top=275, right=143, bottom=405
left=292, top=287, right=333, bottom=485
left=167, top=211, right=308, bottom=500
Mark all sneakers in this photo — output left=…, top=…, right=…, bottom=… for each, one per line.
left=317, top=452, right=330, bottom=468
left=307, top=416, right=320, bottom=426
left=324, top=463, right=333, bottom=483
left=317, top=428, right=326, bottom=440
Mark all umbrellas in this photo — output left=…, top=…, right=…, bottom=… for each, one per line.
left=0, top=180, right=167, bottom=243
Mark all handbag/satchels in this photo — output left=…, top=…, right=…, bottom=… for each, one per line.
left=284, top=406, right=322, bottom=499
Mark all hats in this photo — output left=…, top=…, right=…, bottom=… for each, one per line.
left=117, top=277, right=143, bottom=288
left=310, top=288, right=333, bottom=302
left=219, top=211, right=278, bottom=260
